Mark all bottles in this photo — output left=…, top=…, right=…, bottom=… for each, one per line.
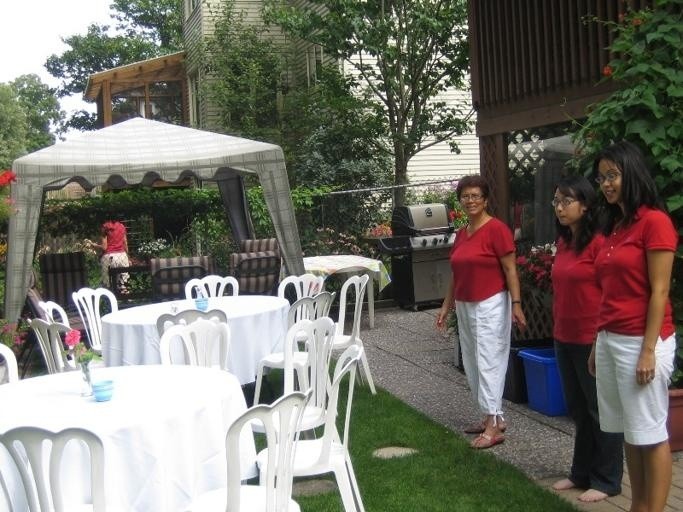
left=194, top=287, right=205, bottom=298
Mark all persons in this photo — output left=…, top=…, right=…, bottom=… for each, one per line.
left=432, top=176, right=529, bottom=449
left=88, top=208, right=133, bottom=303
left=585, top=142, right=678, bottom=511
left=549, top=174, right=627, bottom=500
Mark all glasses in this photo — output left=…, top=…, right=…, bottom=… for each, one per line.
left=550, top=199, right=580, bottom=206
left=458, top=193, right=487, bottom=200
left=593, top=172, right=624, bottom=184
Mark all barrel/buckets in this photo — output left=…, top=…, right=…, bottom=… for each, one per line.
left=666, top=388, right=683, bottom=452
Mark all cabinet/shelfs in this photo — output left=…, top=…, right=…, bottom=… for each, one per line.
left=391, top=255, right=456, bottom=312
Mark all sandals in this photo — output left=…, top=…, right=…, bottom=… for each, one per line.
left=462, top=415, right=509, bottom=435
left=467, top=431, right=507, bottom=449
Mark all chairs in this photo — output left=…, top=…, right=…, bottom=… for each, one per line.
left=0, top=426, right=104, bottom=512
left=157, top=309, right=230, bottom=365
left=27, top=287, right=118, bottom=374
left=225, top=345, right=364, bottom=512
left=185, top=275, right=238, bottom=298
left=253, top=272, right=377, bottom=406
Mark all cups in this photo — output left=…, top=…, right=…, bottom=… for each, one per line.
left=169, top=304, right=179, bottom=314
left=195, top=298, right=209, bottom=312
left=91, top=379, right=115, bottom=402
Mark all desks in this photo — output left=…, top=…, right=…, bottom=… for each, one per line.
left=278, top=255, right=391, bottom=329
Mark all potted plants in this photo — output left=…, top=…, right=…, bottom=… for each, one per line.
left=662, top=324, right=683, bottom=453
